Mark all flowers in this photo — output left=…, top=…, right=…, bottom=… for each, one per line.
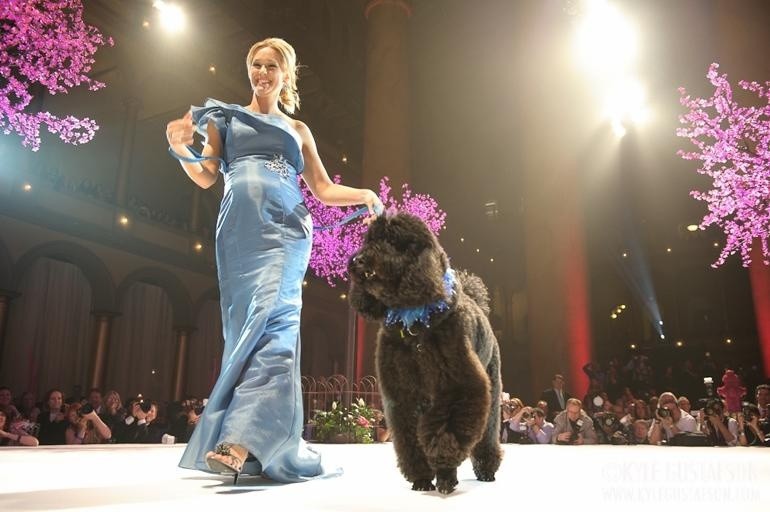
left=308, top=399, right=378, bottom=443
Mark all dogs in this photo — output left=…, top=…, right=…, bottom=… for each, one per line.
left=346, top=211, right=503, bottom=495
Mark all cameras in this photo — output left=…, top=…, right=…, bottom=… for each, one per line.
left=77, top=403, right=94, bottom=416
left=570, top=433, right=579, bottom=441
left=704, top=408, right=713, bottom=416
left=658, top=409, right=670, bottom=416
left=504, top=403, right=515, bottom=414
left=136, top=399, right=151, bottom=412
left=523, top=413, right=532, bottom=420
left=743, top=415, right=753, bottom=424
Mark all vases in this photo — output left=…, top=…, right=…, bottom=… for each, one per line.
left=319, top=431, right=356, bottom=444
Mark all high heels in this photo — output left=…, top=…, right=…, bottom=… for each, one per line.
left=206, top=442, right=243, bottom=485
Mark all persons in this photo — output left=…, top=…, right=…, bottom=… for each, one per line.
left=501, top=351, right=770, bottom=448
left=165, top=39, right=383, bottom=483
left=0, top=387, right=204, bottom=447
